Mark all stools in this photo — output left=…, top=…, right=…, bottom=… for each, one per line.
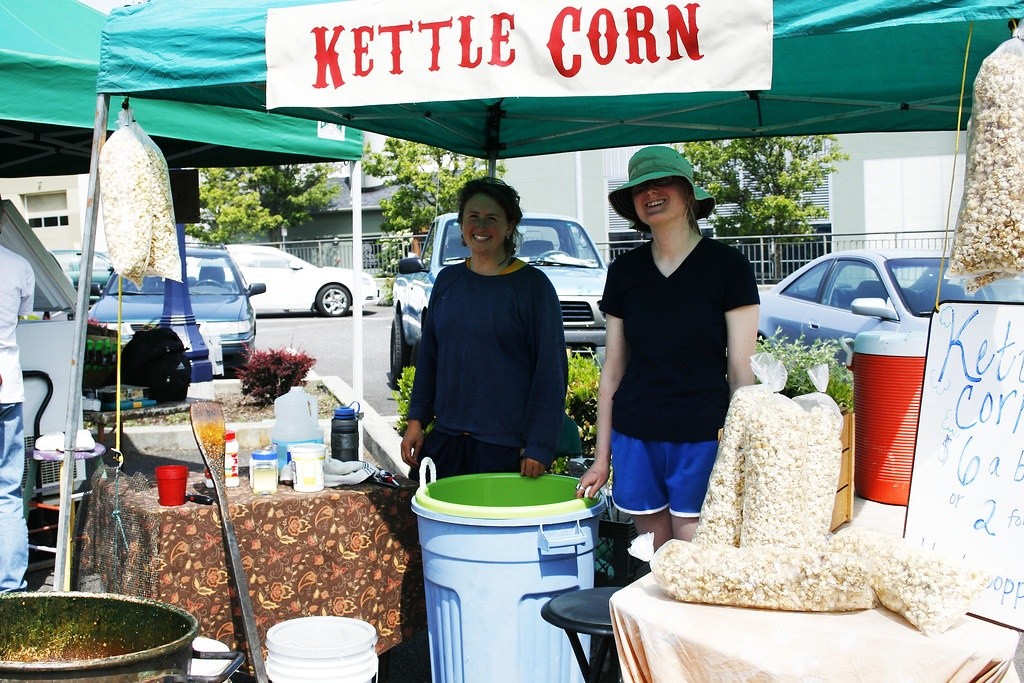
left=541, top=587, right=624, bottom=683
left=22, top=442, right=106, bottom=591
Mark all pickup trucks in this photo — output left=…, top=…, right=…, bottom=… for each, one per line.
left=385, top=214, right=607, bottom=384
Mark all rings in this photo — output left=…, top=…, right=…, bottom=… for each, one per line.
left=575, top=484, right=586, bottom=490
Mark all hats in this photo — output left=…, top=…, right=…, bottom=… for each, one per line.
left=607, top=146, right=715, bottom=231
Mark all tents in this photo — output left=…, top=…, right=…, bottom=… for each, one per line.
left=1, top=0, right=1024, bottom=593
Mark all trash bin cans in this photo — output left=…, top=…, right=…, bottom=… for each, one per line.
left=409, top=457, right=605, bottom=683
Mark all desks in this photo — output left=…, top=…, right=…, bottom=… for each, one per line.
left=609, top=571, right=1024, bottom=683
left=83, top=396, right=224, bottom=472
left=135, top=471, right=427, bottom=675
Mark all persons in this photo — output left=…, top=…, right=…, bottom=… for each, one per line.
left=0, top=196, right=35, bottom=594
left=575, top=145, right=761, bottom=542
left=400, top=176, right=570, bottom=482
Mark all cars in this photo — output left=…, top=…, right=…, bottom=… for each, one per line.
left=87, top=241, right=267, bottom=375
left=755, top=249, right=1024, bottom=345
left=222, top=241, right=385, bottom=318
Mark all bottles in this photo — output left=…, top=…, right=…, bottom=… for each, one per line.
left=331, top=401, right=360, bottom=463
left=203, top=430, right=239, bottom=488
left=271, top=386, right=323, bottom=484
left=253, top=464, right=277, bottom=496
left=87, top=339, right=117, bottom=371
left=249, top=451, right=279, bottom=489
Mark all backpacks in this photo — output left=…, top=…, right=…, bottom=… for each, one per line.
left=119, top=328, right=191, bottom=403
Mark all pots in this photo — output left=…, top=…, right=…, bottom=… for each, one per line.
left=0, top=591, right=245, bottom=683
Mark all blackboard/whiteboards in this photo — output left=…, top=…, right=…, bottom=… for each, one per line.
left=900, top=302, right=1024, bottom=635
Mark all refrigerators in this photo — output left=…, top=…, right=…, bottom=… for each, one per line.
left=0, top=199, right=86, bottom=496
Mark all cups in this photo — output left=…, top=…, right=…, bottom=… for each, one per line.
left=289, top=443, right=327, bottom=492
left=155, top=465, right=188, bottom=506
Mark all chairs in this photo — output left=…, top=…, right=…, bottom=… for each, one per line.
left=855, top=280, right=885, bottom=299
left=940, top=284, right=965, bottom=300
left=518, top=240, right=555, bottom=257
left=447, top=240, right=470, bottom=258
left=199, top=267, right=225, bottom=286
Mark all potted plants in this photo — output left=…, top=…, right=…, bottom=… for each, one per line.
left=757, top=325, right=855, bottom=532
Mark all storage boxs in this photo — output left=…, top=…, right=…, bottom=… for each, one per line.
left=594, top=496, right=652, bottom=587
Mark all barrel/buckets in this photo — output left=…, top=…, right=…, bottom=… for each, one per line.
left=410, top=456, right=606, bottom=683
left=264, top=617, right=378, bottom=683
left=841, top=330, right=930, bottom=507
